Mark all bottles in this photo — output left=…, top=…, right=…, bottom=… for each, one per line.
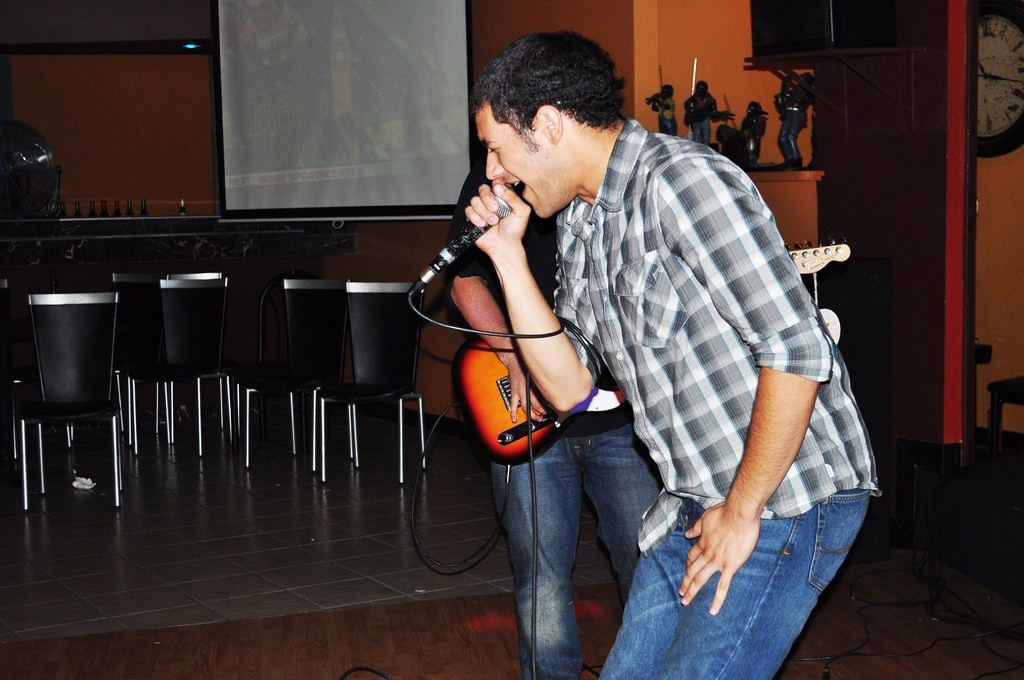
left=87, top=200, right=98, bottom=218
left=57, top=201, right=69, bottom=219
left=125, top=200, right=135, bottom=217
left=177, top=199, right=187, bottom=216
left=73, top=201, right=84, bottom=218
left=113, top=200, right=123, bottom=217
left=138, top=200, right=151, bottom=217
left=99, top=200, right=110, bottom=217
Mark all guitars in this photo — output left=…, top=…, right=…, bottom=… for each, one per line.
left=447, top=236, right=858, bottom=462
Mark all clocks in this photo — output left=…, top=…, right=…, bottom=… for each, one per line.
left=976, top=1, right=1024, bottom=159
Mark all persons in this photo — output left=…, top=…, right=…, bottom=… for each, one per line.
left=650, top=72, right=815, bottom=171
left=473, top=29, right=883, bottom=680
left=433, top=172, right=665, bottom=680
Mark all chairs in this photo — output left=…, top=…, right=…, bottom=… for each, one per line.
left=13, top=265, right=428, bottom=510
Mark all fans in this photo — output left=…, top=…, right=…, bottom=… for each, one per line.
left=0, top=119, right=60, bottom=216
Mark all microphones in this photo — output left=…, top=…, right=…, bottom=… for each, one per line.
left=406, top=184, right=515, bottom=297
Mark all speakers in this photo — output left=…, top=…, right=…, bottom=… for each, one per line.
left=750, top=0, right=896, bottom=57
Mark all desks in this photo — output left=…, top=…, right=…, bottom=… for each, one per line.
left=0, top=216, right=359, bottom=268
